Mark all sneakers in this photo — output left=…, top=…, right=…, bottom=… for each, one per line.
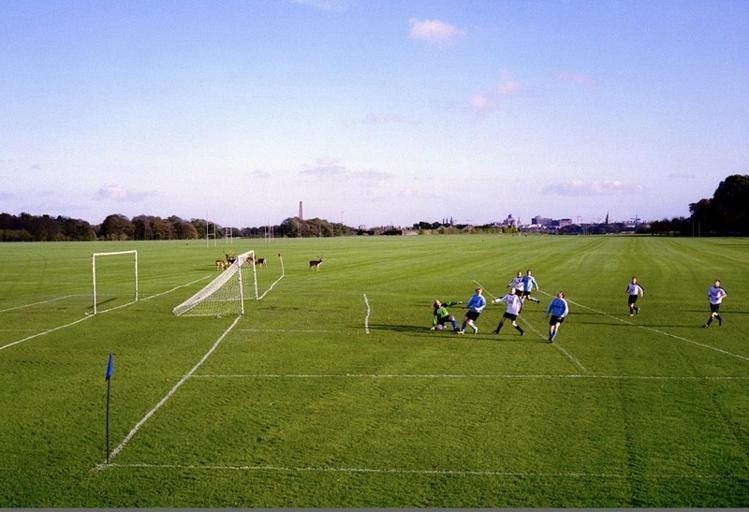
left=701, top=318, right=722, bottom=328
left=629, top=307, right=640, bottom=316
left=520, top=330, right=525, bottom=336
left=452, top=326, right=478, bottom=334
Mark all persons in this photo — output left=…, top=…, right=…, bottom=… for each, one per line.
left=701, top=280, right=728, bottom=328
left=506, top=271, right=524, bottom=299
left=623, top=276, right=646, bottom=317
left=455, top=287, right=487, bottom=334
left=487, top=287, right=526, bottom=336
left=430, top=299, right=463, bottom=331
left=544, top=288, right=569, bottom=343
left=514, top=270, right=540, bottom=313
